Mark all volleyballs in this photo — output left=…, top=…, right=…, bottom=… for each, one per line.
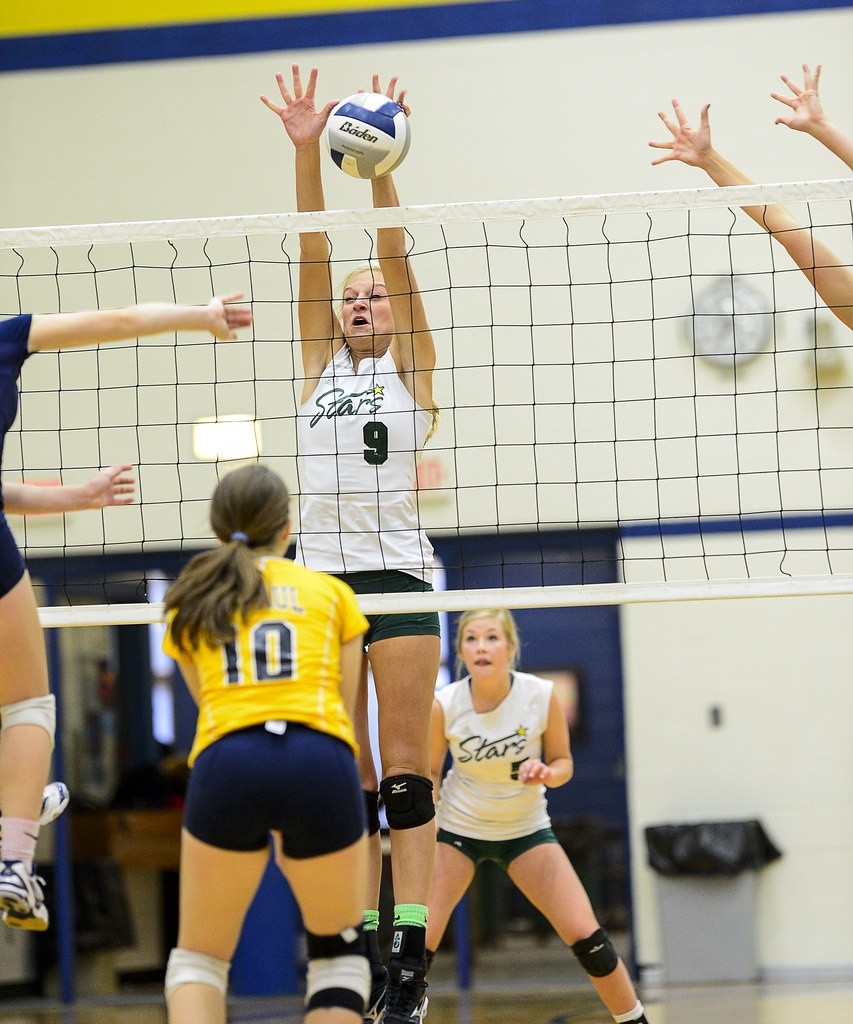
left=323, top=90, right=411, bottom=182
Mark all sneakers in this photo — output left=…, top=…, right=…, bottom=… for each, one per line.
left=0, top=860, right=49, bottom=931
left=382, top=958, right=429, bottom=1024
left=362, top=966, right=389, bottom=1024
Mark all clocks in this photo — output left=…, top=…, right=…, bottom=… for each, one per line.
left=687, top=276, right=771, bottom=369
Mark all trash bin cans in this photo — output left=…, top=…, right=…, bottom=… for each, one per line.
left=643, top=817, right=768, bottom=992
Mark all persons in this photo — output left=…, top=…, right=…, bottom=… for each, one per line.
left=1, top=291, right=252, bottom=935
left=264, top=56, right=445, bottom=1023
left=160, top=462, right=377, bottom=1023
left=646, top=59, right=853, bottom=337
left=425, top=610, right=648, bottom=1023
